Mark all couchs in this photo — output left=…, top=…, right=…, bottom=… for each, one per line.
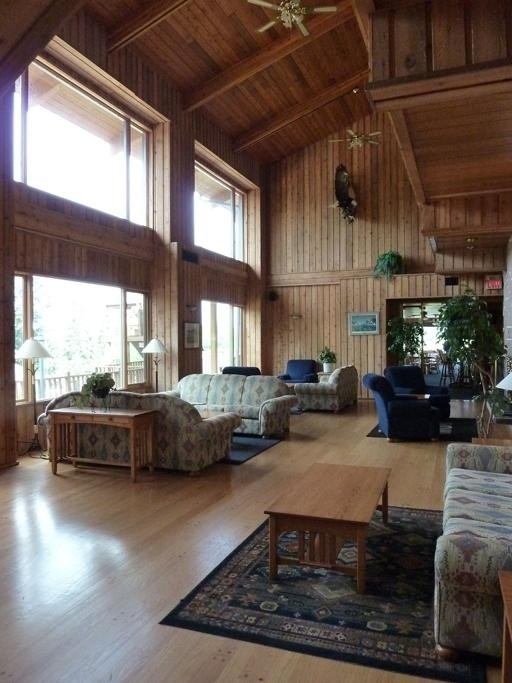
left=433, top=443, right=511, bottom=661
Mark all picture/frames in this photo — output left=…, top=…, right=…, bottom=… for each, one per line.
left=348, top=313, right=379, bottom=336
left=184, top=322, right=200, bottom=349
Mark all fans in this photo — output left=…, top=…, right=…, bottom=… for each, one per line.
left=247, top=0, right=337, bottom=36
left=327, top=88, right=382, bottom=149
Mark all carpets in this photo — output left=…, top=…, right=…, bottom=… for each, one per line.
left=366, top=418, right=477, bottom=443
left=159, top=505, right=488, bottom=682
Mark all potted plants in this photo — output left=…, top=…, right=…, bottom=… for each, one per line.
left=374, top=248, right=402, bottom=282
left=319, top=345, right=336, bottom=372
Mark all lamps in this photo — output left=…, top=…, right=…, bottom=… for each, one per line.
left=350, top=137, right=366, bottom=147
left=15, top=339, right=51, bottom=457
left=275, top=1, right=308, bottom=29
left=495, top=372, right=512, bottom=390
left=141, top=339, right=168, bottom=393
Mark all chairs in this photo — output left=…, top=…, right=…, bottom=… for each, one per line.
left=363, top=366, right=450, bottom=442
left=404, top=349, right=472, bottom=384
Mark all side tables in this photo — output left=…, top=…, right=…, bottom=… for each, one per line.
left=498, top=569, right=512, bottom=682
